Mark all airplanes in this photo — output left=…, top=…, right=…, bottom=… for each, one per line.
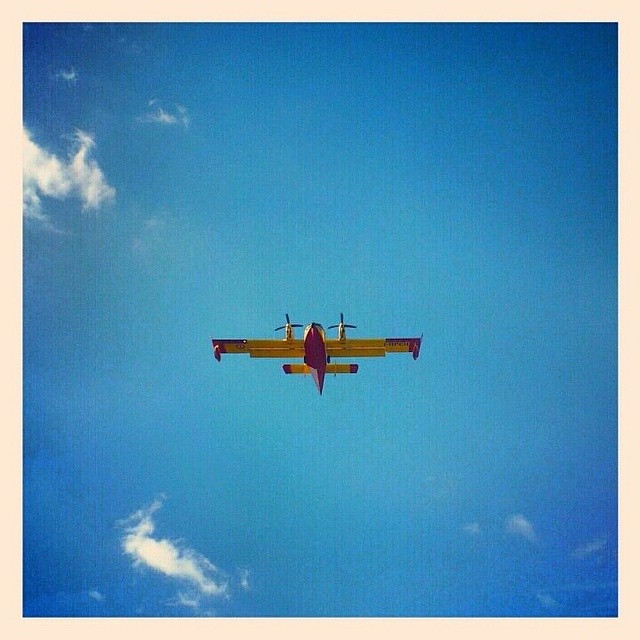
left=212, top=313, right=422, bottom=394
